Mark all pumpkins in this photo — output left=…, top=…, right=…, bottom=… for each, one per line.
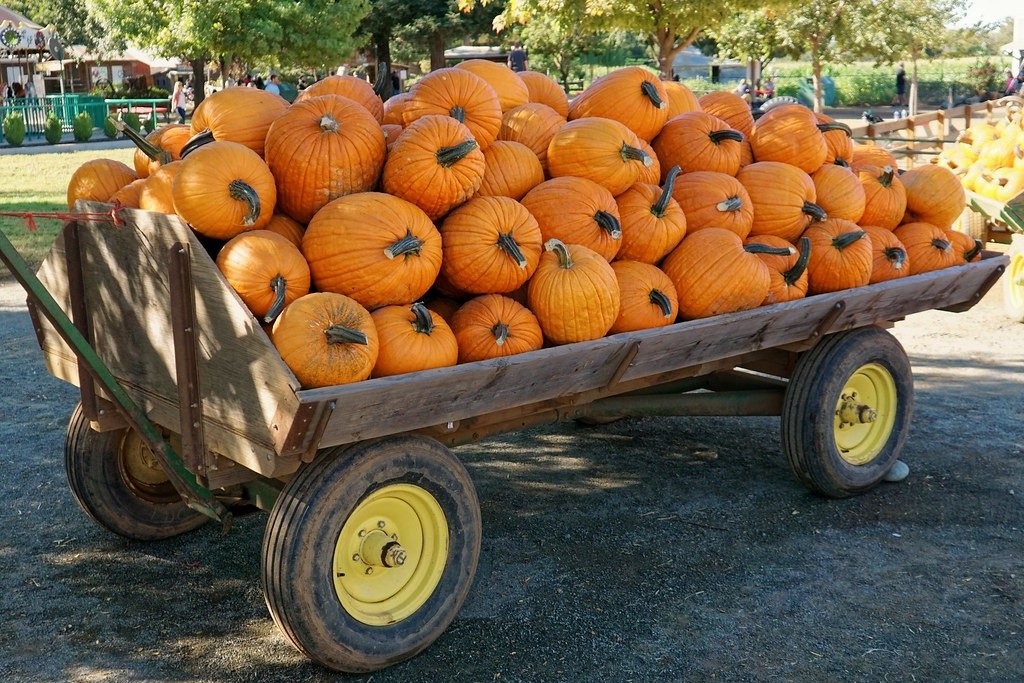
left=935, top=103, right=1024, bottom=204
left=67, top=55, right=982, bottom=386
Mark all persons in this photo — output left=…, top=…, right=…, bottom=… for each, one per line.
left=507, top=43, right=530, bottom=72
left=733, top=78, right=774, bottom=109
left=1004, top=49, right=1024, bottom=98
left=296, top=72, right=340, bottom=90
left=892, top=63, right=910, bottom=107
left=225, top=74, right=264, bottom=90
left=171, top=81, right=187, bottom=124
left=265, top=75, right=280, bottom=96
